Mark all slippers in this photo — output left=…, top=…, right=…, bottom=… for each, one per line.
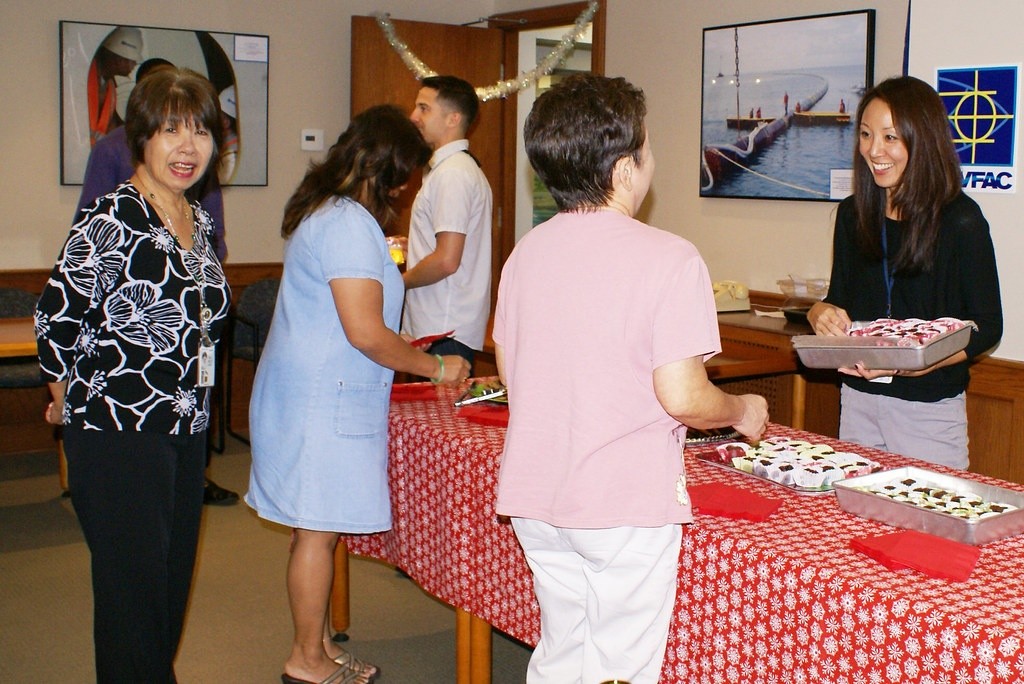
left=282, top=650, right=380, bottom=684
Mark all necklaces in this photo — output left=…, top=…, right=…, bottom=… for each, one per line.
left=135, top=172, right=212, bottom=321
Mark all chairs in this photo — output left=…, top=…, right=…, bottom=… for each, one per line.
left=225, top=277, right=280, bottom=448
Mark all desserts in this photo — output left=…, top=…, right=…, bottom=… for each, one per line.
left=715, top=437, right=880, bottom=486
left=845, top=317, right=969, bottom=346
left=860, top=479, right=1018, bottom=521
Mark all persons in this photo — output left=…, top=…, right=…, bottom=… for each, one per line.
left=492, top=72, right=769, bottom=684
left=33, top=65, right=234, bottom=684
left=806, top=75, right=1003, bottom=471
left=217, top=85, right=238, bottom=185
left=88, top=26, right=143, bottom=150
left=400, top=74, right=494, bottom=383
left=242, top=104, right=471, bottom=684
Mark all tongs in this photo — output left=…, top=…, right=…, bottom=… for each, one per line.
left=454, top=379, right=507, bottom=407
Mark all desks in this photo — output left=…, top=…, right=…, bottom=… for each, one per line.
left=331, top=373, right=1024, bottom=684
left=704, top=305, right=816, bottom=430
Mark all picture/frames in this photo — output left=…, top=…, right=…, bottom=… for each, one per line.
left=699, top=9, right=878, bottom=204
left=58, top=20, right=270, bottom=186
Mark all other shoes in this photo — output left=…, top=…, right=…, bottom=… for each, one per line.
left=201, top=475, right=241, bottom=506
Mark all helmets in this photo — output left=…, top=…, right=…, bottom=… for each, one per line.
left=218, top=83, right=236, bottom=118
left=103, top=27, right=145, bottom=65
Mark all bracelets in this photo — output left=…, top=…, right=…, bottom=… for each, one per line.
left=430, top=354, right=446, bottom=384
